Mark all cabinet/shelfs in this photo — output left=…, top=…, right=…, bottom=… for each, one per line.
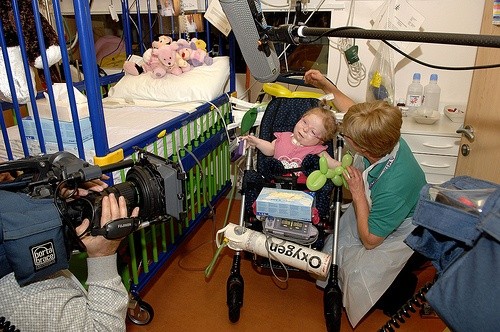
left=253, top=1, right=486, bottom=211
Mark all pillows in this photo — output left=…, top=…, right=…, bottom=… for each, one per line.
left=108, top=54, right=231, bottom=102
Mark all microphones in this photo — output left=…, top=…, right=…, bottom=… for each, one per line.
left=67, top=159, right=134, bottom=182
left=219, top=0, right=280, bottom=84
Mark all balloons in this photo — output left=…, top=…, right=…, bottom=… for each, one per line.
left=306, top=153, right=352, bottom=193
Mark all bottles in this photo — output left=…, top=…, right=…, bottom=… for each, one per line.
left=422, top=74, right=441, bottom=111
left=405, top=73, right=422, bottom=117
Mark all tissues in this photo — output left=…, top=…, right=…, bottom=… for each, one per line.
left=27, top=82, right=91, bottom=123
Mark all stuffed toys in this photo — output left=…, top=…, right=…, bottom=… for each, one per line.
left=123, top=35, right=214, bottom=78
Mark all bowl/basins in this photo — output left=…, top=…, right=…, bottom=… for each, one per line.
left=413, top=109, right=440, bottom=125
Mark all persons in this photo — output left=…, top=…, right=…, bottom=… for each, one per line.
left=304, top=69, right=430, bottom=317
left=0, top=170, right=140, bottom=332
left=242, top=107, right=343, bottom=234
left=162, top=0, right=174, bottom=16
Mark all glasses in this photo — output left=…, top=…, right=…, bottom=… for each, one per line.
left=338, top=131, right=365, bottom=149
left=301, top=117, right=325, bottom=143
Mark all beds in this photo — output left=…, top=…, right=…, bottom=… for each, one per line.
left=0, top=0, right=235, bottom=325
